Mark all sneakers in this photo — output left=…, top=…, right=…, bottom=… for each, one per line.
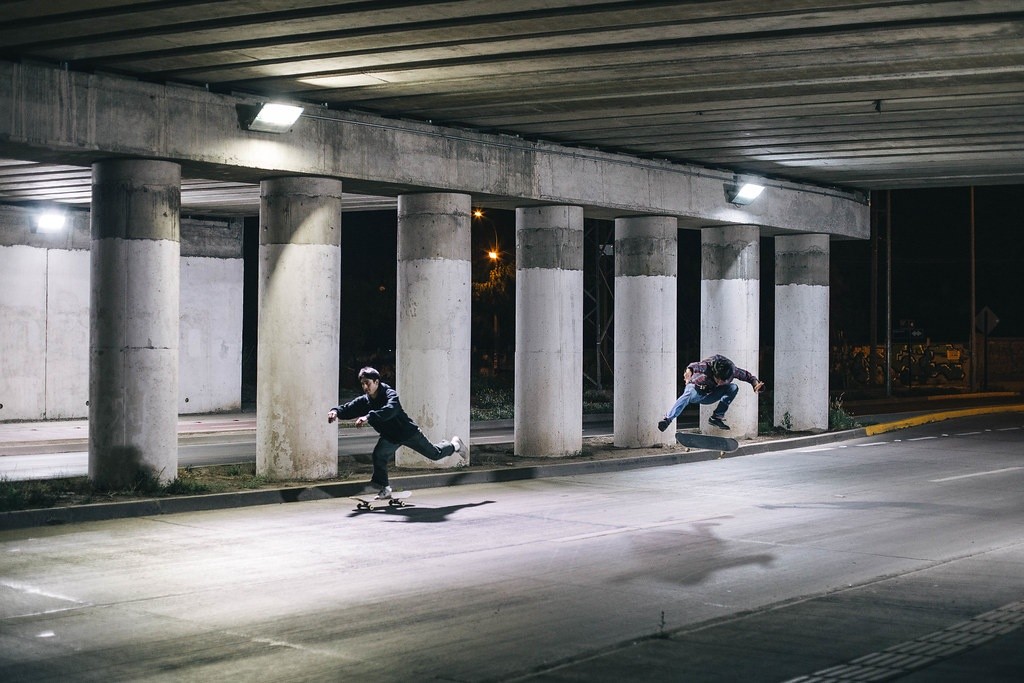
left=374, top=487, right=392, bottom=499
left=708, top=416, right=730, bottom=430
left=658, top=419, right=669, bottom=432
left=451, top=436, right=469, bottom=460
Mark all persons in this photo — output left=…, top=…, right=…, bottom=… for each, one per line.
left=327, top=367, right=469, bottom=500
left=658, top=354, right=766, bottom=432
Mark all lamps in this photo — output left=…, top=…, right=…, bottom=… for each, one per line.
left=242, top=101, right=301, bottom=134
left=729, top=181, right=766, bottom=208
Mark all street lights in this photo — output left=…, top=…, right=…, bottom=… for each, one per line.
left=474, top=208, right=499, bottom=377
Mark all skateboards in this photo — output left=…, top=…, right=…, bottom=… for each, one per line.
left=675, top=431, right=740, bottom=455
left=347, top=491, right=413, bottom=507
